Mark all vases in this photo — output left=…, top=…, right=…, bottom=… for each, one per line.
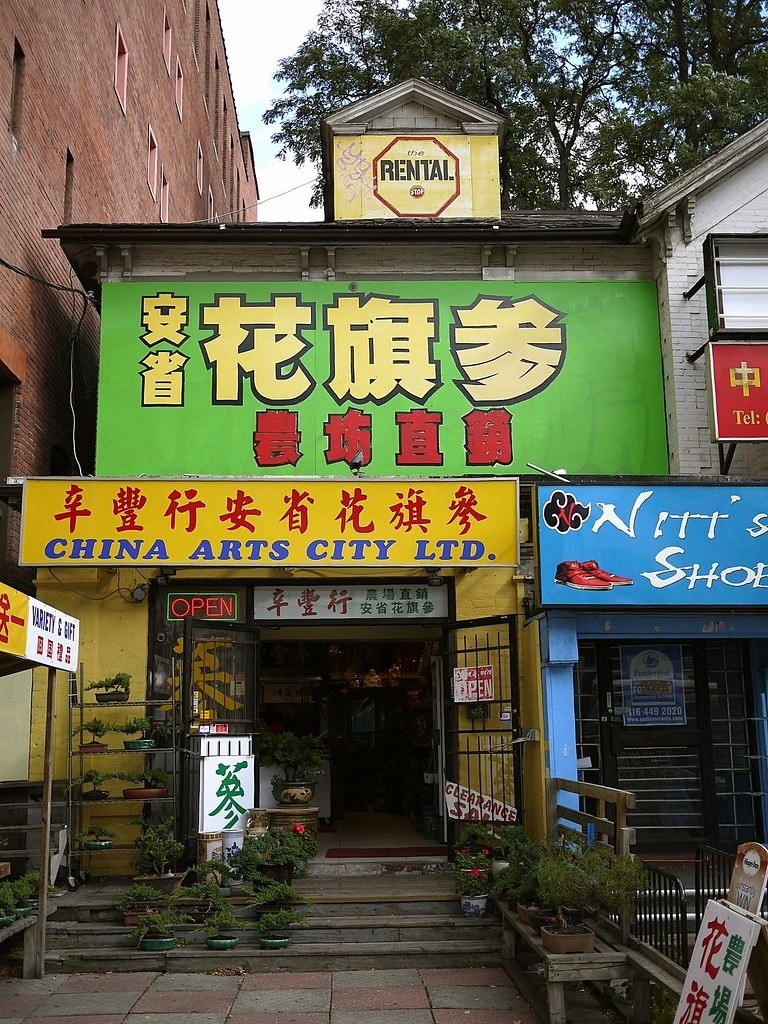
left=540, top=926, right=595, bottom=954
left=459, top=894, right=489, bottom=919
left=275, top=853, right=308, bottom=879
left=248, top=807, right=319, bottom=838
left=459, top=868, right=491, bottom=885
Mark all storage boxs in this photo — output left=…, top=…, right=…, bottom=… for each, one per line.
left=423, top=810, right=437, bottom=839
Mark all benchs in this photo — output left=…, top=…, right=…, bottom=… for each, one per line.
left=494, top=895, right=650, bottom=1024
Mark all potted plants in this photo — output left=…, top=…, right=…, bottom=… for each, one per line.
left=450, top=823, right=579, bottom=935
left=118, top=826, right=321, bottom=952
left=68, top=672, right=186, bottom=893
left=256, top=721, right=328, bottom=805
left=0, top=870, right=63, bottom=928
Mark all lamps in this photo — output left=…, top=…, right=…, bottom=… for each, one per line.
left=426, top=568, right=443, bottom=586
left=349, top=449, right=366, bottom=477
left=155, top=568, right=176, bottom=587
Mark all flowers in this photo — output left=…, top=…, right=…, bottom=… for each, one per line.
left=456, top=867, right=490, bottom=894
left=455, top=849, right=494, bottom=869
left=536, top=828, right=650, bottom=932
left=280, top=822, right=320, bottom=858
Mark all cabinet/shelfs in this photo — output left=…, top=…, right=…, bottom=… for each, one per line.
left=66, top=655, right=183, bottom=893
left=260, top=645, right=433, bottom=809
